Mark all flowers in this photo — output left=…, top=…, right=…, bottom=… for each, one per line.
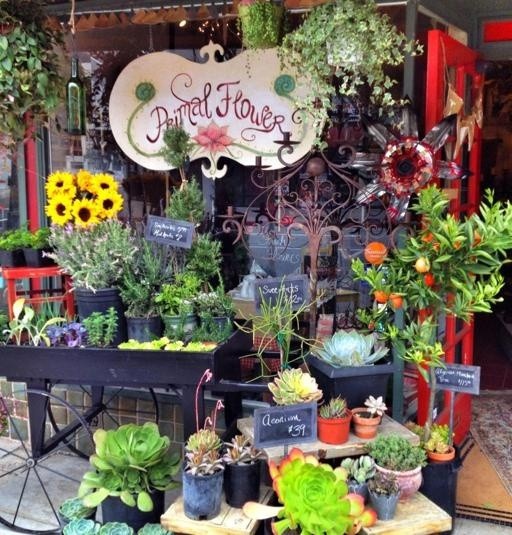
left=42, top=168, right=125, bottom=226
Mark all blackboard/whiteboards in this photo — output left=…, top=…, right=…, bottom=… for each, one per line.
left=428, top=363, right=481, bottom=396
left=253, top=274, right=310, bottom=313
left=145, top=214, right=194, bottom=249
left=254, top=401, right=317, bottom=450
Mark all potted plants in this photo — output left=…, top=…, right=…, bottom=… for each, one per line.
left=0, top=0, right=83, bottom=178
left=0, top=126, right=236, bottom=350
left=347, top=183, right=512, bottom=515
left=58, top=421, right=181, bottom=535
left=235, top=1, right=425, bottom=156
left=183, top=328, right=456, bottom=533
